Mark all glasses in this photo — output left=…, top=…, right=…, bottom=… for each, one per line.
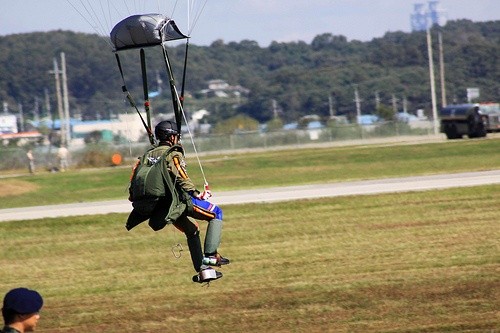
left=177, top=133, right=181, bottom=139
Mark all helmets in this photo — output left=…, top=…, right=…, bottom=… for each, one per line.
left=154, top=121, right=180, bottom=140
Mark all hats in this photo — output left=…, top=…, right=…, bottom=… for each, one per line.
left=1, top=286, right=43, bottom=314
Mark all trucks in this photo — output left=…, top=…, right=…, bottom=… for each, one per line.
left=440, top=105, right=500, bottom=138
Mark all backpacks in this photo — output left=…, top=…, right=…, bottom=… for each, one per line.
left=130, top=145, right=187, bottom=215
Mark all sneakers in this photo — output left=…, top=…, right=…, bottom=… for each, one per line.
left=192, top=271, right=222, bottom=282
left=203, top=250, right=229, bottom=265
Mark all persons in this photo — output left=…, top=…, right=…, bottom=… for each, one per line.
left=129, top=120, right=230, bottom=283
left=0, top=288, right=43, bottom=333
